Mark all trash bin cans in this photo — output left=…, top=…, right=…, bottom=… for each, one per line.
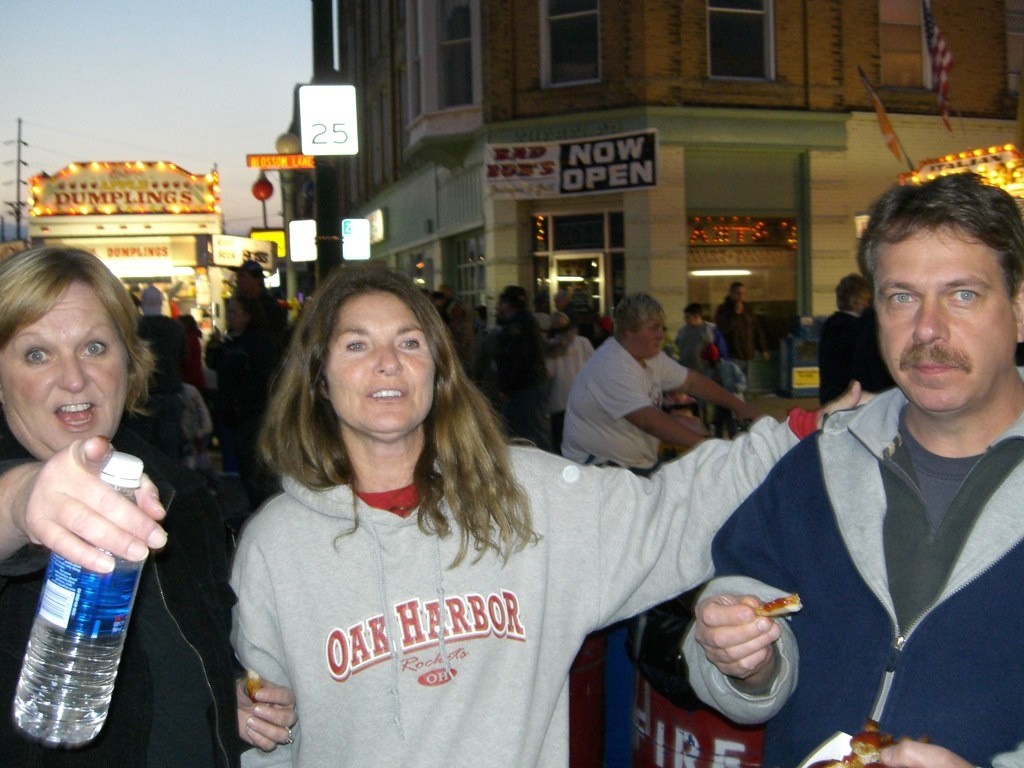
left=569, top=617, right=766, bottom=768
left=778, top=312, right=821, bottom=400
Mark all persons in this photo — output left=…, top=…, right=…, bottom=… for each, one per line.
left=430, top=285, right=554, bottom=452
left=114, top=261, right=289, bottom=513
left=623, top=171, right=1024, bottom=768
left=228, top=266, right=878, bottom=768
left=676, top=303, right=729, bottom=378
left=545, top=311, right=594, bottom=459
left=698, top=343, right=748, bottom=442
left=715, top=282, right=770, bottom=439
left=566, top=286, right=614, bottom=351
left=561, top=293, right=758, bottom=478
left=1, top=245, right=298, bottom=768
left=818, top=273, right=897, bottom=407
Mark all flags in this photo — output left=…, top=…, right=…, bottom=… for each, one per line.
left=919, top=0, right=959, bottom=134
left=859, top=75, right=905, bottom=164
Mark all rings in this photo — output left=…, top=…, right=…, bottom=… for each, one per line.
left=285, top=727, right=293, bottom=746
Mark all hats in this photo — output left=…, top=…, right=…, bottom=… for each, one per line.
left=228, top=260, right=264, bottom=277
left=701, top=344, right=720, bottom=361
left=597, top=318, right=613, bottom=334
left=549, top=311, right=571, bottom=329
left=141, top=285, right=163, bottom=317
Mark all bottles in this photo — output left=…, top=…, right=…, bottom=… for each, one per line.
left=11, top=451, right=145, bottom=746
